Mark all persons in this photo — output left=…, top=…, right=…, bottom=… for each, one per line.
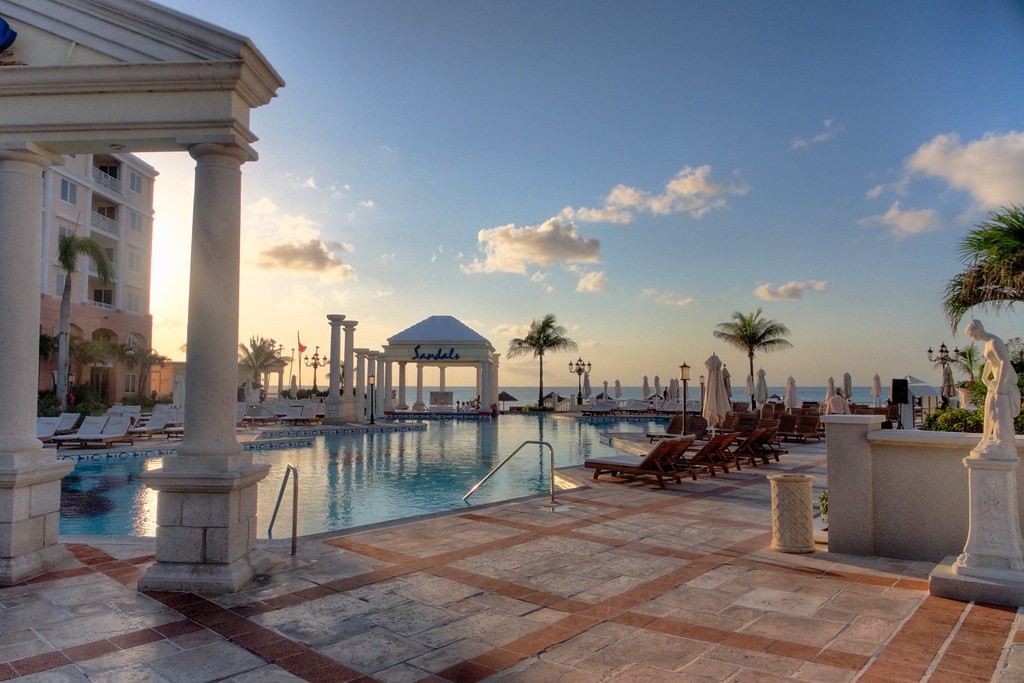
left=826, top=387, right=851, bottom=415
left=965, top=319, right=1021, bottom=452
left=457, top=400, right=473, bottom=413
left=661, top=386, right=669, bottom=408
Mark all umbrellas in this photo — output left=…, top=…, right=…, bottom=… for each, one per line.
left=615, top=380, right=623, bottom=399
left=746, top=367, right=769, bottom=418
left=603, top=380, right=608, bottom=401
left=653, top=375, right=661, bottom=410
left=842, top=372, right=852, bottom=399
left=642, top=375, right=650, bottom=400
left=581, top=370, right=591, bottom=404
left=289, top=375, right=298, bottom=399
left=870, top=373, right=881, bottom=407
left=825, top=376, right=836, bottom=401
left=171, top=373, right=185, bottom=411
left=668, top=378, right=679, bottom=401
left=704, top=352, right=733, bottom=437
left=902, top=375, right=926, bottom=385
left=595, top=392, right=613, bottom=400
left=498, top=391, right=518, bottom=410
left=537, top=392, right=566, bottom=402
left=785, top=374, right=797, bottom=415
left=938, top=364, right=959, bottom=398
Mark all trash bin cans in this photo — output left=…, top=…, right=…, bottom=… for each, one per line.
left=767, top=474, right=817, bottom=553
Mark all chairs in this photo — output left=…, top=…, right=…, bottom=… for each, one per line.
left=37, top=398, right=319, bottom=449
left=582, top=395, right=900, bottom=494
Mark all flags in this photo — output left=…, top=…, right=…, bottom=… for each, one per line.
left=299, top=342, right=307, bottom=353
